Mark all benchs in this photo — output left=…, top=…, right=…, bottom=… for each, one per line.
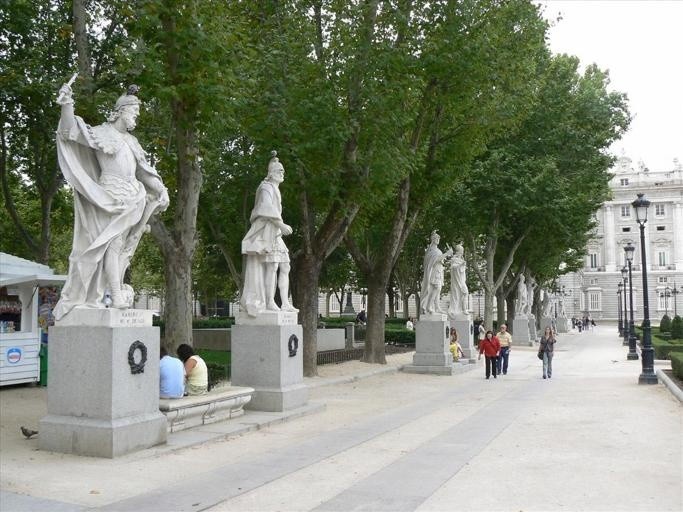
left=159, top=385, right=254, bottom=433
left=454, top=358, right=471, bottom=375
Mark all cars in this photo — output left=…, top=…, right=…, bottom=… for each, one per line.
left=152, top=309, right=159, bottom=318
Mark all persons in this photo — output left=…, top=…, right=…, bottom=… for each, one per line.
left=356, top=310, right=367, bottom=326
left=447, top=244, right=475, bottom=319
left=160, top=345, right=187, bottom=400
left=420, top=229, right=453, bottom=315
left=406, top=316, right=413, bottom=331
left=176, top=344, right=208, bottom=396
left=570, top=316, right=596, bottom=332
left=450, top=321, right=512, bottom=379
left=540, top=327, right=556, bottom=379
left=514, top=273, right=566, bottom=319
left=51, top=82, right=171, bottom=322
left=239, top=150, right=300, bottom=317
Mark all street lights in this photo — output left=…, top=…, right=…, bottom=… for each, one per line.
left=614, top=242, right=638, bottom=362
left=631, top=191, right=658, bottom=385
left=659, top=281, right=682, bottom=319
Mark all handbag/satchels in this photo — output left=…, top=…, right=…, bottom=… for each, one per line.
left=538, top=350, right=544, bottom=360
